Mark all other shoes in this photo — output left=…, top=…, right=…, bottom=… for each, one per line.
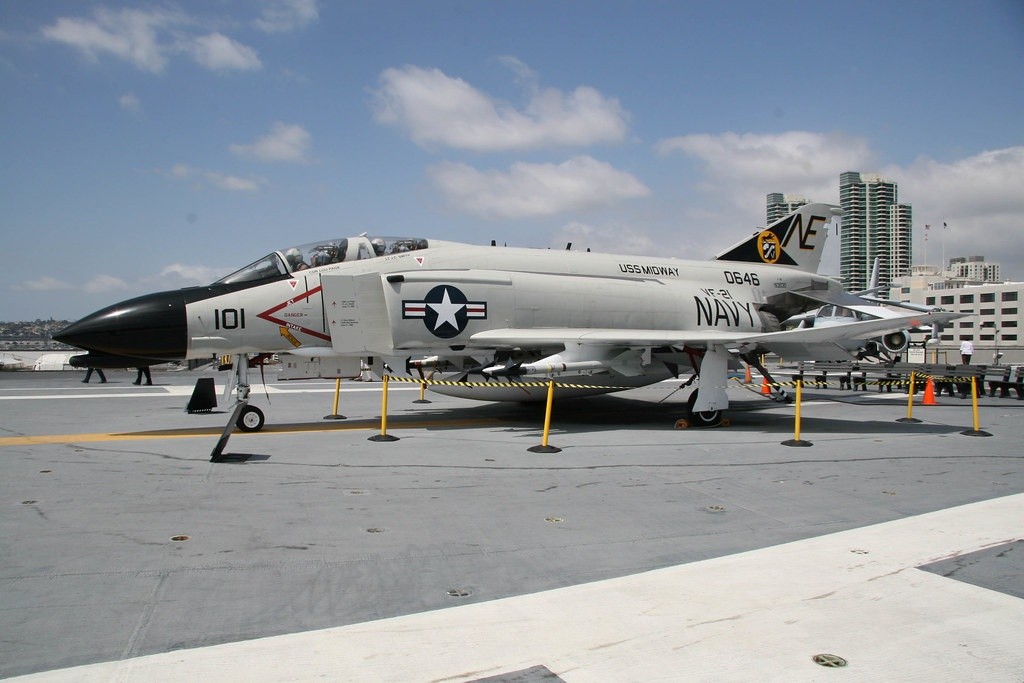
left=98, top=380, right=107, bottom=383
left=133, top=381, right=140, bottom=385
left=81, top=380, right=88, bottom=383
left=143, top=381, right=152, bottom=385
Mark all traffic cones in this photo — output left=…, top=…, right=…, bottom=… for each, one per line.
left=760, top=368, right=772, bottom=394
left=920, top=378, right=938, bottom=405
left=743, top=364, right=753, bottom=383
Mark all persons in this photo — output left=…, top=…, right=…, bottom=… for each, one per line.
left=81, top=367, right=107, bottom=383
left=960, top=337, right=973, bottom=365
left=133, top=366, right=152, bottom=385
left=283, top=249, right=310, bottom=272
left=371, top=238, right=389, bottom=256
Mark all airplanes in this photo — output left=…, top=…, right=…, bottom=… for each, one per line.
left=54, top=203, right=974, bottom=463
